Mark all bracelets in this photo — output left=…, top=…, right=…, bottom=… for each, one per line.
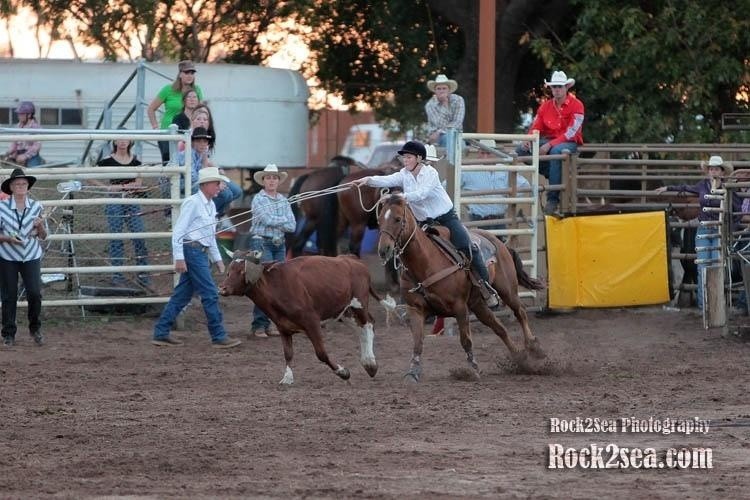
left=121, top=183, right=125, bottom=191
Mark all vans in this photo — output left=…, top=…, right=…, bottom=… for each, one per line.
left=365, top=140, right=532, bottom=241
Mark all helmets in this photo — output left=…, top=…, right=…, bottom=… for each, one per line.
left=13, top=101, right=36, bottom=115
left=397, top=141, right=428, bottom=161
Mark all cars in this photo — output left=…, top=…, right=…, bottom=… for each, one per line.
left=301, top=223, right=379, bottom=260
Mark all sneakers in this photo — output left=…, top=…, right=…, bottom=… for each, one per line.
left=218, top=217, right=240, bottom=234
left=474, top=276, right=501, bottom=310
left=147, top=333, right=183, bottom=348
left=210, top=335, right=242, bottom=349
left=541, top=195, right=560, bottom=215
left=265, top=323, right=283, bottom=338
left=248, top=327, right=269, bottom=341
left=1, top=333, right=15, bottom=349
left=29, top=329, right=44, bottom=346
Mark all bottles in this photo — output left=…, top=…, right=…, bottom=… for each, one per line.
left=56, top=181, right=83, bottom=193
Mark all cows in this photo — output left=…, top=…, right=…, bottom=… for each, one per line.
left=218, top=243, right=408, bottom=386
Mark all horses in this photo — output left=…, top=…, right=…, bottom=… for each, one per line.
left=284, top=165, right=367, bottom=260
left=610, top=152, right=750, bottom=308
left=378, top=192, right=544, bottom=383
left=325, top=155, right=402, bottom=258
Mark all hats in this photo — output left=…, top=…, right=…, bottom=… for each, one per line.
left=250, top=161, right=289, bottom=190
left=176, top=58, right=197, bottom=73
left=468, top=137, right=503, bottom=153
left=543, top=69, right=577, bottom=89
left=426, top=73, right=459, bottom=95
left=189, top=165, right=232, bottom=189
left=699, top=154, right=731, bottom=174
left=189, top=125, right=213, bottom=140
left=1, top=168, right=37, bottom=194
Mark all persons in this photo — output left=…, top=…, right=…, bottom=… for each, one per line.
left=148, top=60, right=264, bottom=349
left=424, top=74, right=467, bottom=148
left=6, top=100, right=45, bottom=169
left=0, top=168, right=50, bottom=347
left=84, top=126, right=152, bottom=288
left=252, top=164, right=296, bottom=338
left=735, top=160, right=750, bottom=318
left=441, top=137, right=532, bottom=242
left=654, top=155, right=742, bottom=310
left=350, top=141, right=500, bottom=324
left=517, top=70, right=585, bottom=215
